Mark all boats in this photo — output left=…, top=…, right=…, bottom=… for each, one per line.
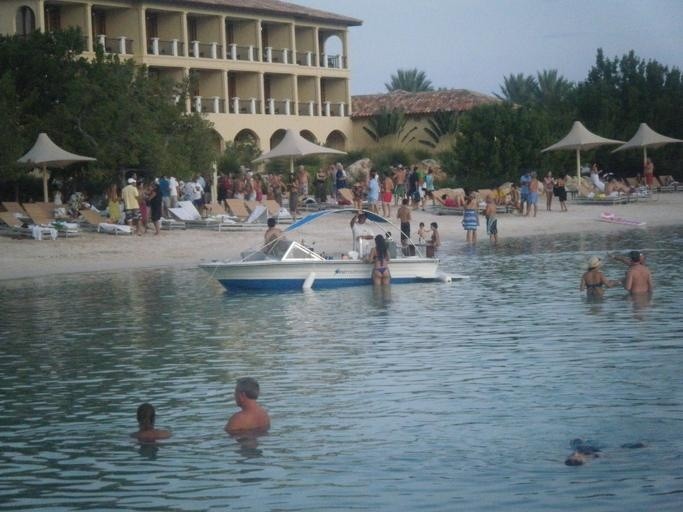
left=199, top=208, right=443, bottom=294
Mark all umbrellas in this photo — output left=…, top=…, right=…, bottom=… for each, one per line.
left=610, top=123, right=683, bottom=171
left=15, top=132, right=97, bottom=202
left=541, top=120, right=625, bottom=185
left=252, top=128, right=348, bottom=175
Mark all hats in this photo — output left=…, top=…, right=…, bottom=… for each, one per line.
left=128, top=178, right=136, bottom=184
left=583, top=255, right=607, bottom=271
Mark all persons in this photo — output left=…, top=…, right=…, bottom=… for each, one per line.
left=561, top=452, right=588, bottom=465
left=581, top=256, right=614, bottom=296
left=622, top=251, right=653, bottom=293
left=224, top=376, right=270, bottom=432
left=461, top=195, right=498, bottom=241
left=217, top=164, right=348, bottom=212
left=108, top=171, right=213, bottom=236
left=351, top=160, right=568, bottom=219
left=129, top=403, right=172, bottom=441
left=590, top=158, right=654, bottom=196
left=264, top=199, right=441, bottom=285
